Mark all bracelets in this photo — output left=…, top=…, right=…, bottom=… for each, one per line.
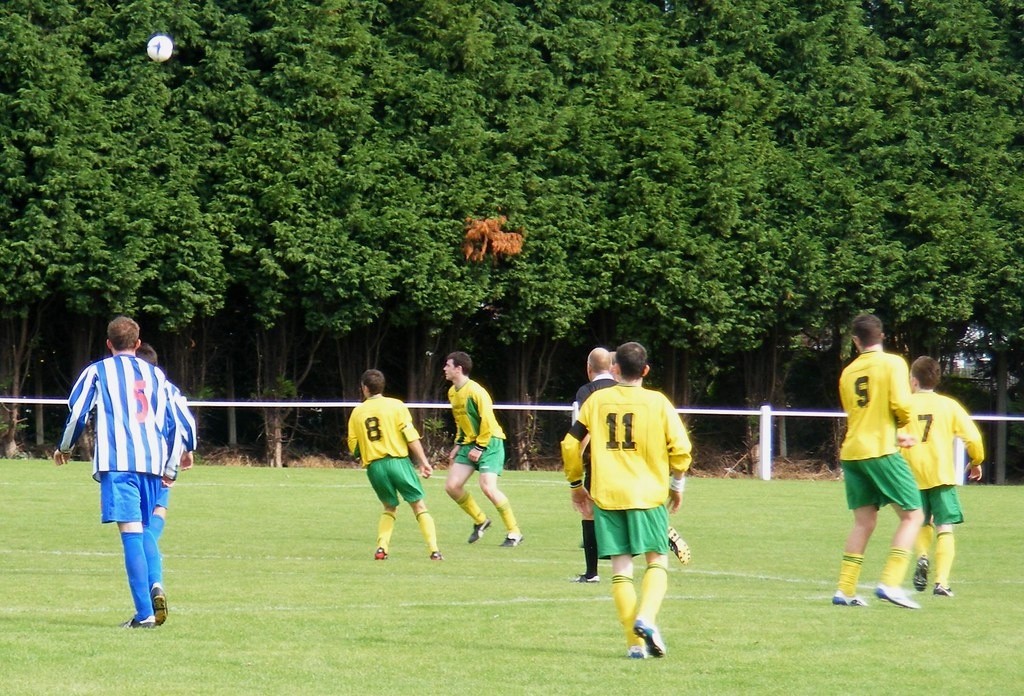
left=670, top=476, right=685, bottom=493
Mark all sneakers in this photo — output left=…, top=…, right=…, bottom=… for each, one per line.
left=632, top=617, right=666, bottom=658
left=832, top=591, right=869, bottom=607
left=934, top=582, right=954, bottom=596
left=120, top=614, right=155, bottom=628
left=500, top=533, right=525, bottom=548
left=914, top=555, right=929, bottom=593
left=666, top=526, right=692, bottom=565
left=430, top=550, right=445, bottom=560
left=567, top=573, right=601, bottom=585
left=375, top=548, right=388, bottom=561
left=150, top=583, right=168, bottom=624
left=627, top=646, right=647, bottom=660
left=468, top=517, right=492, bottom=544
left=875, top=582, right=920, bottom=609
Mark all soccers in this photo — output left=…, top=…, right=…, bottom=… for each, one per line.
left=146, top=35, right=174, bottom=62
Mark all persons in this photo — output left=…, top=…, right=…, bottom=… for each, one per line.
left=561, top=342, right=692, bottom=659
left=135, top=345, right=197, bottom=540
left=443, top=352, right=525, bottom=547
left=347, top=369, right=447, bottom=561
left=833, top=313, right=925, bottom=610
left=569, top=347, right=692, bottom=583
left=53, top=316, right=182, bottom=630
left=897, top=356, right=985, bottom=597
